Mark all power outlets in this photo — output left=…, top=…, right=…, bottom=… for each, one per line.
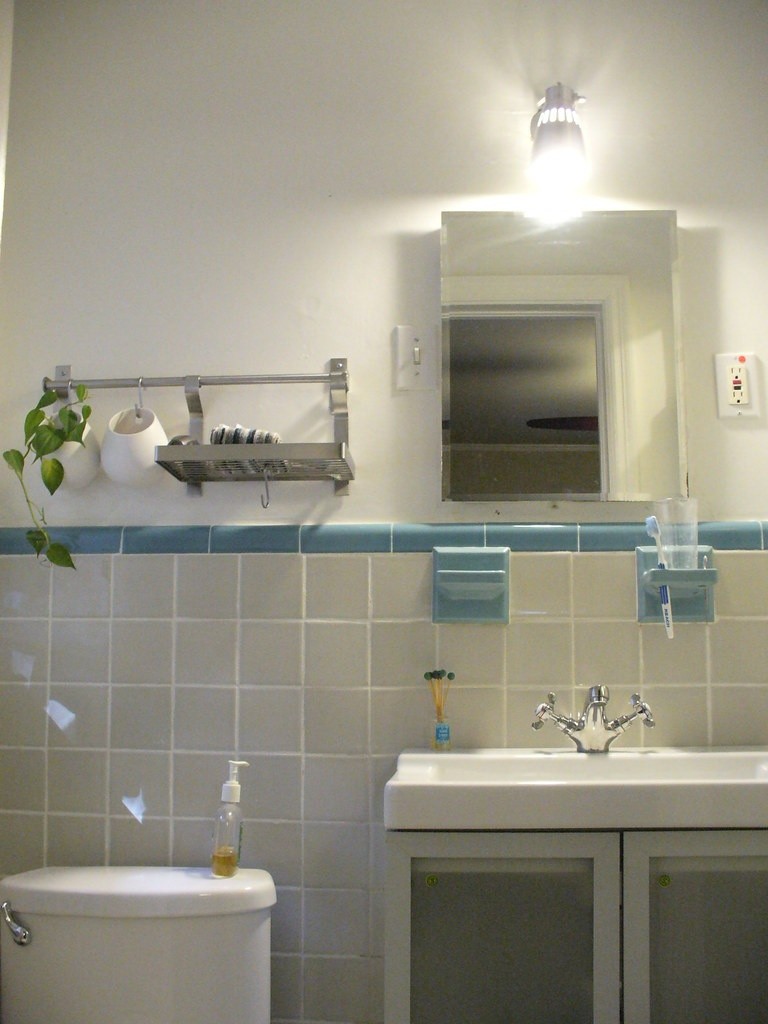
left=715, top=352, right=760, bottom=418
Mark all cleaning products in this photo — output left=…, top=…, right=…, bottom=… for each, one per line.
left=211, top=758, right=250, bottom=876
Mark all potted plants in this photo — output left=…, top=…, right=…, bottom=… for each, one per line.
left=3, top=384, right=101, bottom=571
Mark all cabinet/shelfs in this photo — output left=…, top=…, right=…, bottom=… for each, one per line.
left=382, top=830, right=768, bottom=1024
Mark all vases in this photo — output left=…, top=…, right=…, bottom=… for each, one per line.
left=101, top=408, right=169, bottom=488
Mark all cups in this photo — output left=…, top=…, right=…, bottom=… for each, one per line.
left=656, top=498, right=699, bottom=570
left=41, top=411, right=100, bottom=490
left=432, top=717, right=452, bottom=752
left=100, top=408, right=168, bottom=487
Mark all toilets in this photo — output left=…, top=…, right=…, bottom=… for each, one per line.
left=0, top=864, right=278, bottom=1024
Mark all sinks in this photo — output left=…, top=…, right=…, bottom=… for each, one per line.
left=380, top=746, right=768, bottom=829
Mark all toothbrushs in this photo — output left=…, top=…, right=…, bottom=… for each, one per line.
left=647, top=516, right=677, bottom=642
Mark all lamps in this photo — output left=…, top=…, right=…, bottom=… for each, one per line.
left=527, top=82, right=589, bottom=187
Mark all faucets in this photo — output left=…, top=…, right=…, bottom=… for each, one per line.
left=530, top=682, right=659, bottom=752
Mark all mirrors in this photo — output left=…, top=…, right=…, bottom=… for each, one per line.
left=439, top=208, right=691, bottom=503
